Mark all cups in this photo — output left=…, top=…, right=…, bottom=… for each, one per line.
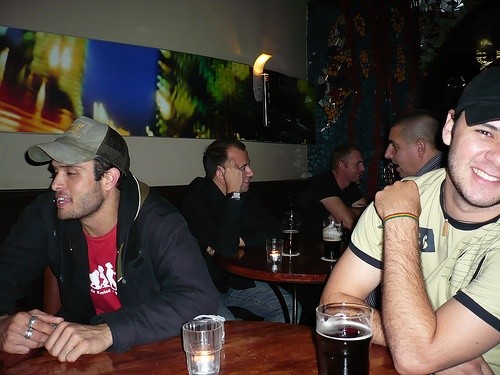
left=315, top=302, right=374, bottom=375
left=322, top=219, right=343, bottom=262
left=266, top=238, right=283, bottom=269
left=282, top=212, right=301, bottom=255
left=182, top=320, right=222, bottom=375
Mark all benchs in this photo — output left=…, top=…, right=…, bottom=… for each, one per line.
left=0, top=177, right=379, bottom=326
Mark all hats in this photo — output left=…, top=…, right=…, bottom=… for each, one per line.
left=454, top=68, right=500, bottom=127
left=27, top=116, right=130, bottom=176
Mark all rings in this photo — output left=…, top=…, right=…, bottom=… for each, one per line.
left=25, top=329, right=34, bottom=338
left=28, top=315, right=37, bottom=328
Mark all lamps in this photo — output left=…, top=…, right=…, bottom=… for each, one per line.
left=253, top=53, right=272, bottom=127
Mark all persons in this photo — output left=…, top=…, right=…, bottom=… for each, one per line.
left=295, top=144, right=369, bottom=229
left=385, top=109, right=445, bottom=178
left=0, top=117, right=220, bottom=362
left=320, top=58, right=500, bottom=375
left=176, top=136, right=302, bottom=323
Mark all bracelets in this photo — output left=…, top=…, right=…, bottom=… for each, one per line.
left=382, top=213, right=419, bottom=226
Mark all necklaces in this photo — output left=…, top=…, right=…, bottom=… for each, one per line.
left=441, top=184, right=480, bottom=236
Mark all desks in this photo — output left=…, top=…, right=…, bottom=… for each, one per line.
left=0, top=320, right=397, bottom=375
left=219, top=237, right=332, bottom=326
